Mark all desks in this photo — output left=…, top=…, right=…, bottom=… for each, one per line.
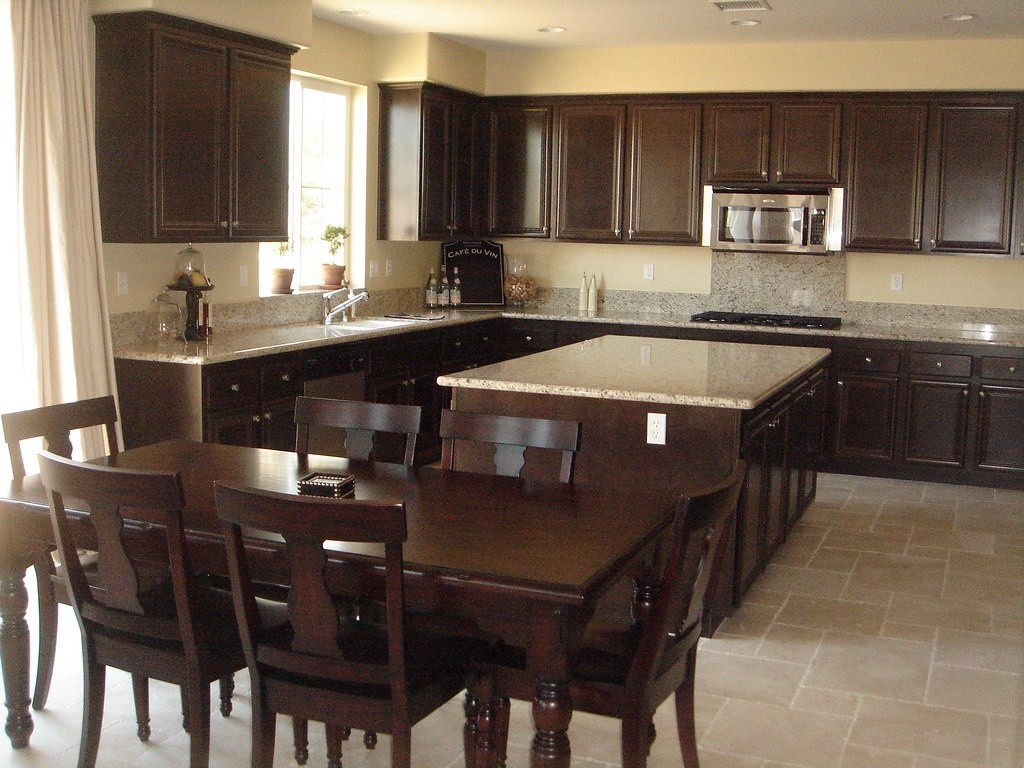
left=0, top=438, right=678, bottom=768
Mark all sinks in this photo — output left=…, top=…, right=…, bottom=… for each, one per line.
left=328, top=320, right=416, bottom=330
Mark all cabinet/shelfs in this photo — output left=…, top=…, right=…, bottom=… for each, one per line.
left=376, top=80, right=1024, bottom=262
left=202, top=324, right=606, bottom=474
left=92, top=10, right=300, bottom=243
left=737, top=351, right=1024, bottom=610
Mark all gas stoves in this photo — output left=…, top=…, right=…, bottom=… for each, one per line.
left=690, top=311, right=841, bottom=331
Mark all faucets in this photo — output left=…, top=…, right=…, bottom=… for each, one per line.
left=322, top=286, right=370, bottom=325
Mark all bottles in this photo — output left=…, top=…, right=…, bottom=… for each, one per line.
left=438, top=265, right=449, bottom=307
left=578, top=272, right=587, bottom=311
left=505, top=253, right=536, bottom=302
left=197, top=291, right=212, bottom=337
left=449, top=267, right=460, bottom=307
left=425, top=268, right=438, bottom=309
left=589, top=271, right=597, bottom=312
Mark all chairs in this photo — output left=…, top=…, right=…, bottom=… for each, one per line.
left=0, top=395, right=748, bottom=768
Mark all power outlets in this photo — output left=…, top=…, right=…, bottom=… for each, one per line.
left=644, top=263, right=654, bottom=279
left=891, top=274, right=902, bottom=291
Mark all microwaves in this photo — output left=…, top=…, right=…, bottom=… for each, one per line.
left=702, top=184, right=844, bottom=256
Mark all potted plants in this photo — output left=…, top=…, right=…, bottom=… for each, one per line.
left=320, top=225, right=350, bottom=286
left=270, top=241, right=294, bottom=289
